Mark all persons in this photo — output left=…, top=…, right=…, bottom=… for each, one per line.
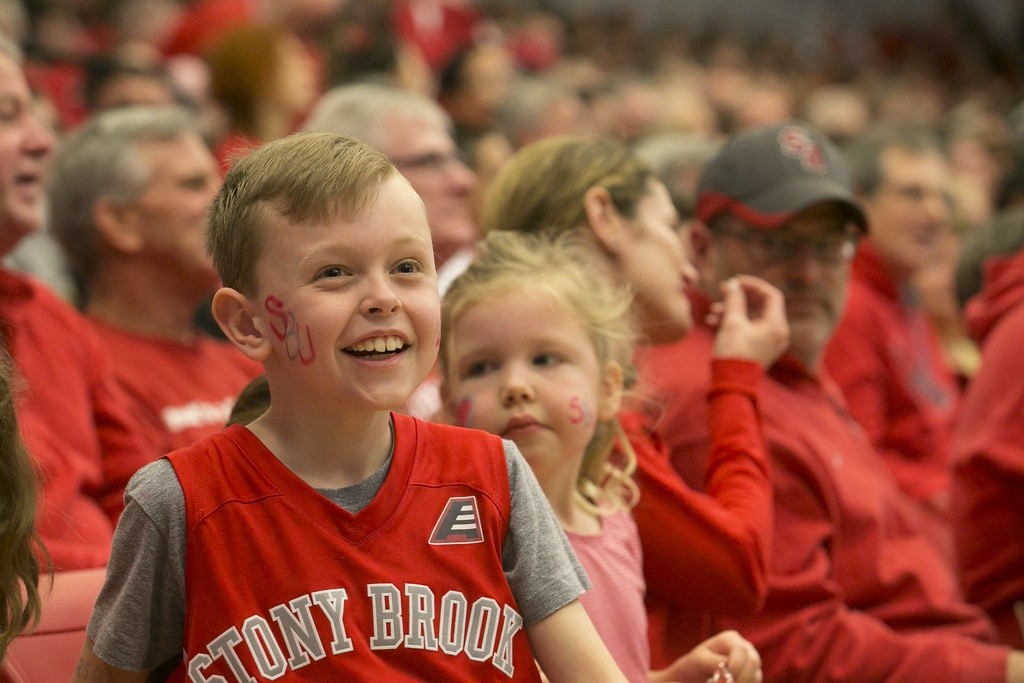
left=440, top=229, right=761, bottom=683
left=74, top=131, right=630, bottom=683
left=0, top=0, right=1024, bottom=683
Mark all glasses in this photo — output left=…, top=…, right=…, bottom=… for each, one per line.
left=701, top=219, right=861, bottom=264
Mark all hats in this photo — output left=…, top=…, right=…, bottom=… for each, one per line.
left=692, top=115, right=874, bottom=236
